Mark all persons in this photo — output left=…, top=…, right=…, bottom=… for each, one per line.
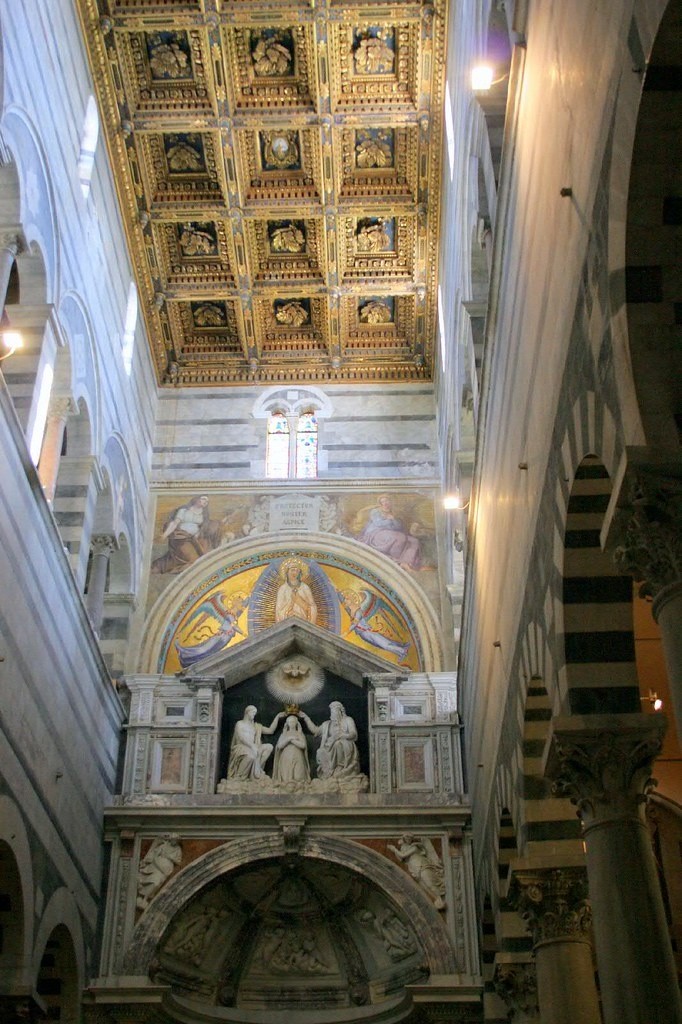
left=224, top=700, right=363, bottom=784
left=128, top=832, right=185, bottom=909
left=387, top=832, right=448, bottom=915
